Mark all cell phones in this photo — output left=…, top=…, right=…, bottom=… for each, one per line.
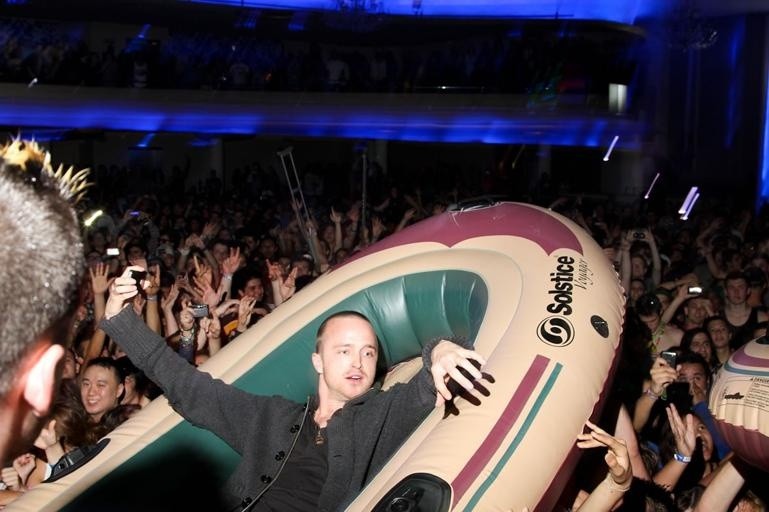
left=662, top=351, right=677, bottom=369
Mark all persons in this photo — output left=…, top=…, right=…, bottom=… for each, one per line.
left=1, top=129, right=769, bottom=511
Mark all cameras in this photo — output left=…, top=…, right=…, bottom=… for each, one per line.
left=189, top=304, right=209, bottom=319
left=132, top=271, right=146, bottom=291
left=688, top=286, right=703, bottom=295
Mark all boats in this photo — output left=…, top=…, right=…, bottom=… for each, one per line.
left=707, top=325, right=769, bottom=469
left=8, top=195, right=633, bottom=512
